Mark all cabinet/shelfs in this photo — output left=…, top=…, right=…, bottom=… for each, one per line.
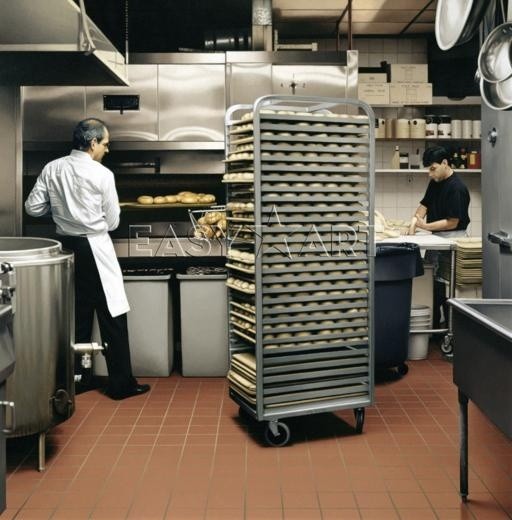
left=227, top=92, right=377, bottom=449
left=373, top=131, right=482, bottom=175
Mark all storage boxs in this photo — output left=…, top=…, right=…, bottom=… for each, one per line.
left=358, top=64, right=433, bottom=106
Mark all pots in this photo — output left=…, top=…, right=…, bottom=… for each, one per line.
left=433, top=0, right=512, bottom=114
left=0, top=234, right=76, bottom=440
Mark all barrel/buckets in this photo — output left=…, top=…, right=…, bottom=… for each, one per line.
left=407, top=304, right=431, bottom=361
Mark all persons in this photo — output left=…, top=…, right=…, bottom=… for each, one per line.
left=25, top=117, right=151, bottom=401
left=413, top=145, right=471, bottom=341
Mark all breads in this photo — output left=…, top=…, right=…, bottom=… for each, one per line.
left=227, top=109, right=371, bottom=350
left=137, top=190, right=227, bottom=239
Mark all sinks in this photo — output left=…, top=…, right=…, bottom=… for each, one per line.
left=447, top=297, right=512, bottom=342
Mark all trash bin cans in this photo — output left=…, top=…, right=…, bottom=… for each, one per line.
left=374, top=242, right=424, bottom=375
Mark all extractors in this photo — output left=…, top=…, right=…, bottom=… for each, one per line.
left=0, top=0, right=129, bottom=89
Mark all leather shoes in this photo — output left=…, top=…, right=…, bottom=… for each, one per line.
left=75, top=376, right=108, bottom=395
left=114, top=383, right=151, bottom=401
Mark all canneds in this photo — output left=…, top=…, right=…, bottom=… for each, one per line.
left=423, top=115, right=437, bottom=138
left=437, top=115, right=452, bottom=138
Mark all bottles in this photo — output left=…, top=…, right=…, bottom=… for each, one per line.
left=390, top=146, right=400, bottom=169
left=450, top=143, right=467, bottom=168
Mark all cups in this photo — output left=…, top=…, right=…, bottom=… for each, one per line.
left=399, top=152, right=420, bottom=169
left=450, top=119, right=481, bottom=138
